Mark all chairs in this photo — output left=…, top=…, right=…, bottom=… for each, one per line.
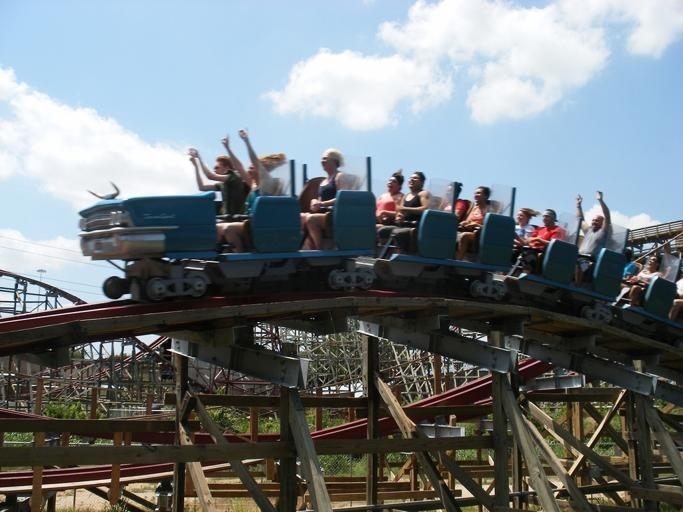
left=299, top=177, right=679, bottom=288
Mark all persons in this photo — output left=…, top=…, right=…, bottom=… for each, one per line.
left=186, top=129, right=683, bottom=321
